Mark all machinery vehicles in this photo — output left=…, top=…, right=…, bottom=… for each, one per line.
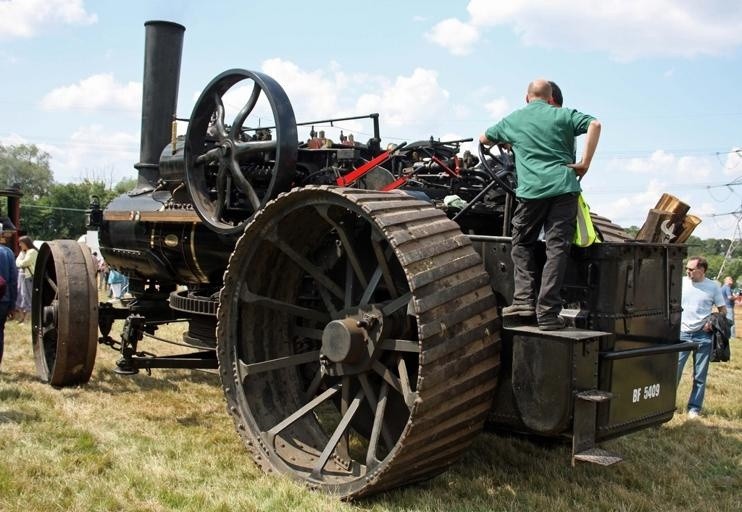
left=31, top=18, right=707, bottom=503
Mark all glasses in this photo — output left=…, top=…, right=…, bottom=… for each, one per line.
left=685, top=267, right=697, bottom=271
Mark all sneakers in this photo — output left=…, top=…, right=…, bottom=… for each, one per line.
left=539, top=316, right=566, bottom=329
left=689, top=410, right=698, bottom=417
left=501, top=303, right=534, bottom=317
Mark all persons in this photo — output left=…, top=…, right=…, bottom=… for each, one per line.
left=719, top=275, right=739, bottom=340
left=480, top=81, right=599, bottom=329
left=92, top=252, right=132, bottom=301
left=0, top=222, right=19, bottom=367
left=674, top=255, right=726, bottom=417
left=14, top=235, right=39, bottom=324
